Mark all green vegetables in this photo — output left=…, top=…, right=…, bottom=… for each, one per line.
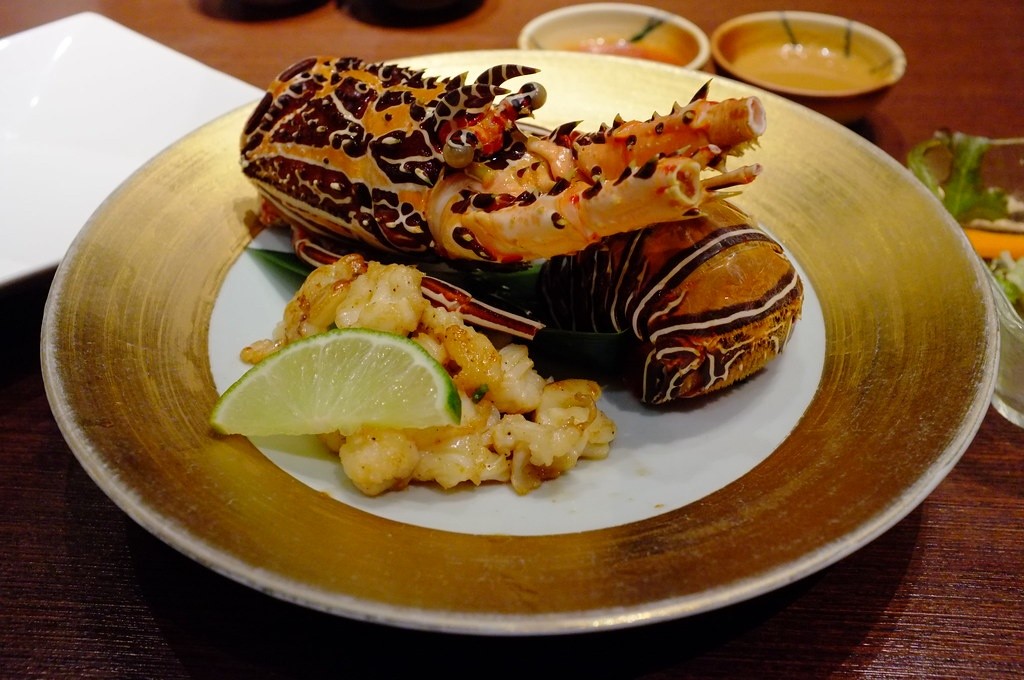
left=904, top=126, right=1024, bottom=326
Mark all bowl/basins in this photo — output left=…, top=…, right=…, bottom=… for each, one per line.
left=910, top=135, right=1024, bottom=430
left=714, top=13, right=906, bottom=121
left=516, top=4, right=711, bottom=69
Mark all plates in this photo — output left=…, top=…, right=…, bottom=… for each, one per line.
left=41, top=48, right=998, bottom=637
left=0, top=11, right=267, bottom=282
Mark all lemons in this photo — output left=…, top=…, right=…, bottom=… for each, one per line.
left=208, top=326, right=461, bottom=436
